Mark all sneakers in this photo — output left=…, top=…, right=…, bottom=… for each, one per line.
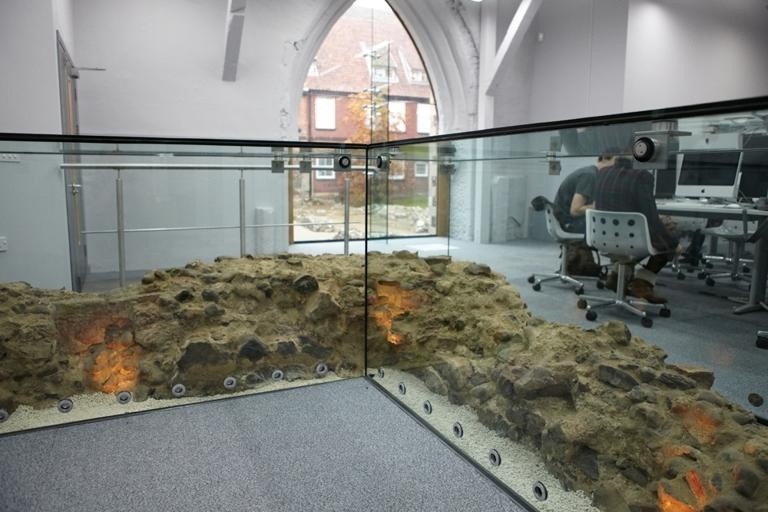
left=605, top=272, right=667, bottom=305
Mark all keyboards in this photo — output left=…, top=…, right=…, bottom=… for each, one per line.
left=662, top=202, right=725, bottom=209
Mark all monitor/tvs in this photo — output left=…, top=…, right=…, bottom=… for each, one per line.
left=674, top=149, right=744, bottom=198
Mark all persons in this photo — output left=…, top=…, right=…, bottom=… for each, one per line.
left=553, top=156, right=617, bottom=234
left=594, top=155, right=670, bottom=305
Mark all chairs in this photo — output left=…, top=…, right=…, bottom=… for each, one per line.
left=527, top=195, right=751, bottom=328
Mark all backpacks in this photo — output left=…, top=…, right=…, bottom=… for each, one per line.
left=558, top=241, right=609, bottom=277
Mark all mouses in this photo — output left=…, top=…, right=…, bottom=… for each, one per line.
left=726, top=202, right=742, bottom=209
